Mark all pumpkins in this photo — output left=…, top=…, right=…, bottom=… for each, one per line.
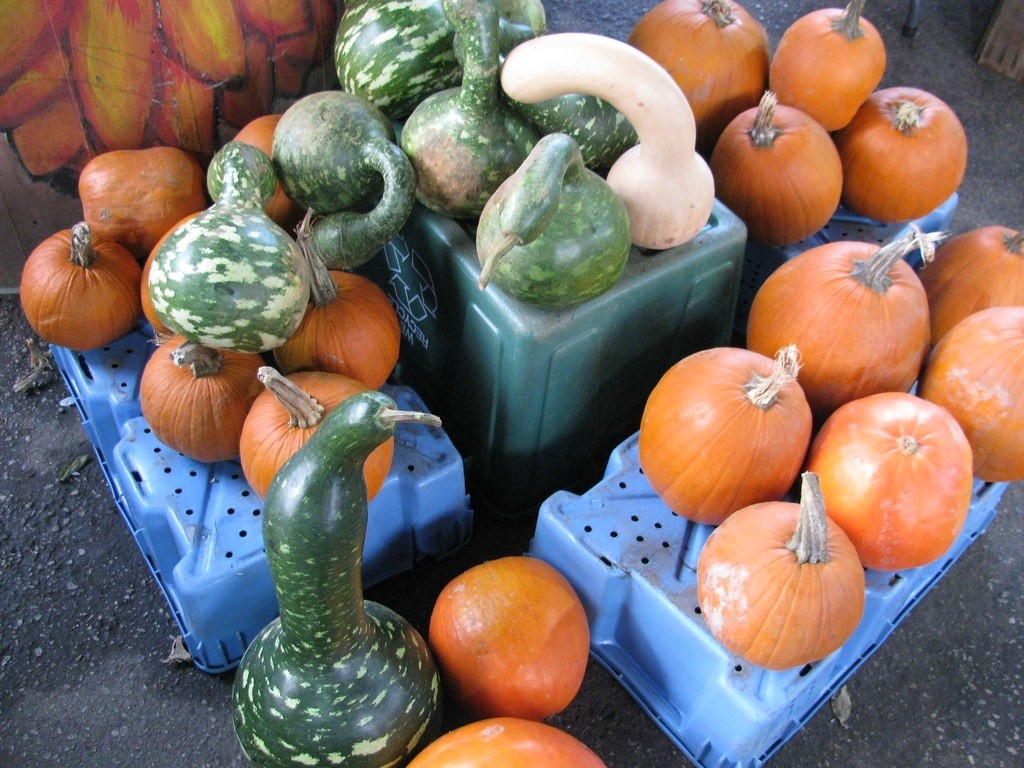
left=18, top=1, right=1024, bottom=768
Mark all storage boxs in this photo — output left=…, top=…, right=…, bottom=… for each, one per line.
left=42, top=121, right=1012, bottom=768
